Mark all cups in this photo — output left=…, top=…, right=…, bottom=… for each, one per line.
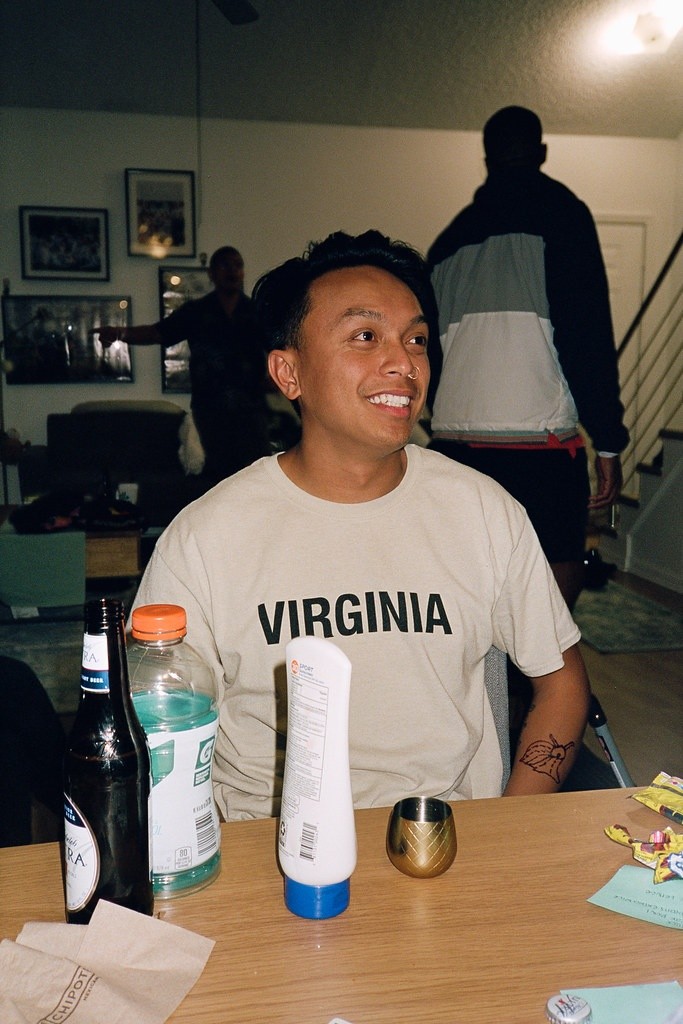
left=386, top=797, right=458, bottom=878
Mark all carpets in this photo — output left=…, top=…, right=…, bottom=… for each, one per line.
left=0, top=619, right=84, bottom=714
left=570, top=580, right=682, bottom=653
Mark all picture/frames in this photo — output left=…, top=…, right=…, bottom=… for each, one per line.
left=124, top=165, right=198, bottom=261
left=0, top=295, right=137, bottom=385
left=158, top=265, right=216, bottom=394
left=18, top=204, right=111, bottom=284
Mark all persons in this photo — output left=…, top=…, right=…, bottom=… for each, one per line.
left=90, top=245, right=265, bottom=498
left=426, top=106, right=631, bottom=615
left=122, top=229, right=590, bottom=822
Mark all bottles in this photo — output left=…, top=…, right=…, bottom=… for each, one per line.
left=277, top=633, right=358, bottom=920
left=59, top=597, right=155, bottom=925
left=126, top=605, right=222, bottom=903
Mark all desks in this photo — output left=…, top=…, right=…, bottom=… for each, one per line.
left=0, top=788, right=683, bottom=1024
